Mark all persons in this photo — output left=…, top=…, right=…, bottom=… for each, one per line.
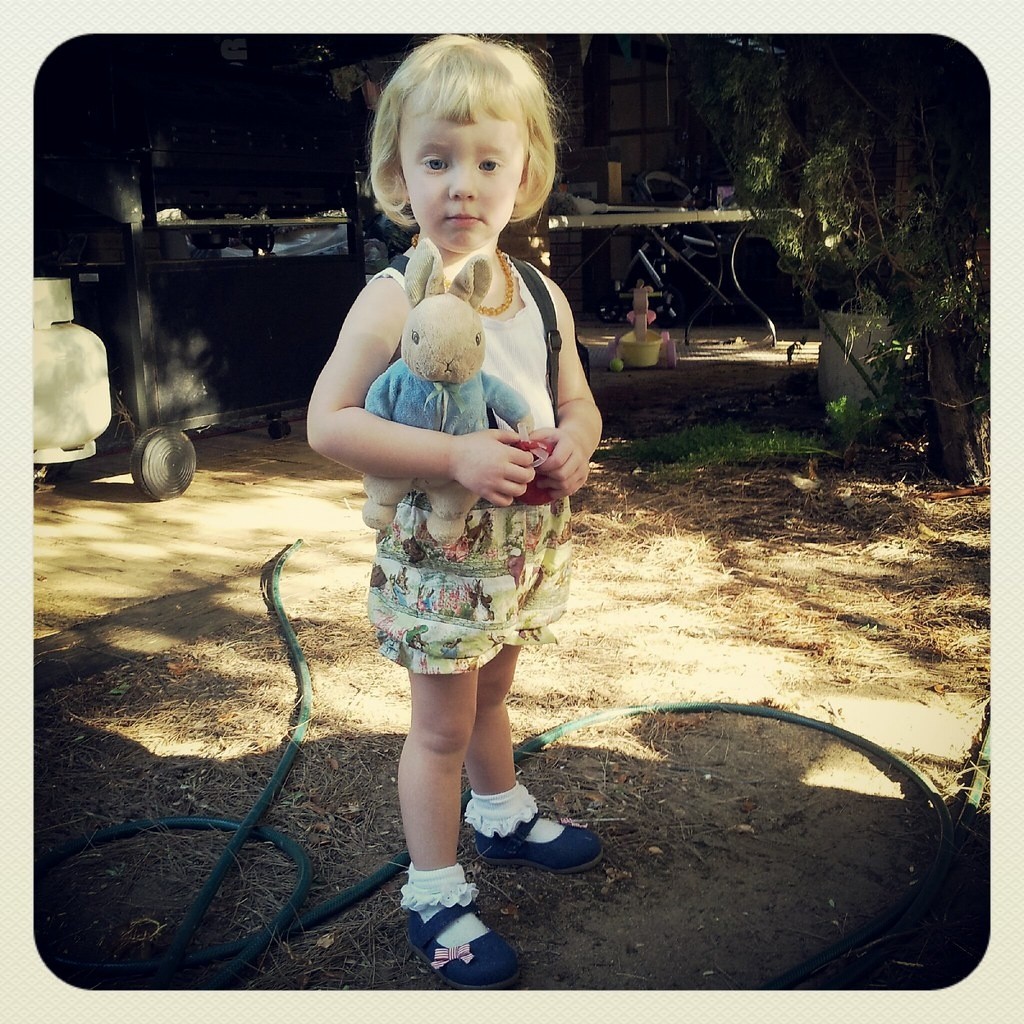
left=308, top=33, right=603, bottom=990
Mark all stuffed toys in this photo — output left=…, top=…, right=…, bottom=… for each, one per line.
left=361, top=238, right=537, bottom=545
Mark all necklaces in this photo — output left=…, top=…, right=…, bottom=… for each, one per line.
left=410, top=234, right=514, bottom=314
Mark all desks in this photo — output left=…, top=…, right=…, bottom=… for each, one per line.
left=160, top=218, right=351, bottom=255
left=546, top=208, right=808, bottom=349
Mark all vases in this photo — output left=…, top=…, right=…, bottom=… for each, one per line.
left=816, top=308, right=905, bottom=415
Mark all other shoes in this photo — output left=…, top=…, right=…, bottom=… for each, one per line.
left=475, top=813, right=604, bottom=874
left=406, top=902, right=520, bottom=989
